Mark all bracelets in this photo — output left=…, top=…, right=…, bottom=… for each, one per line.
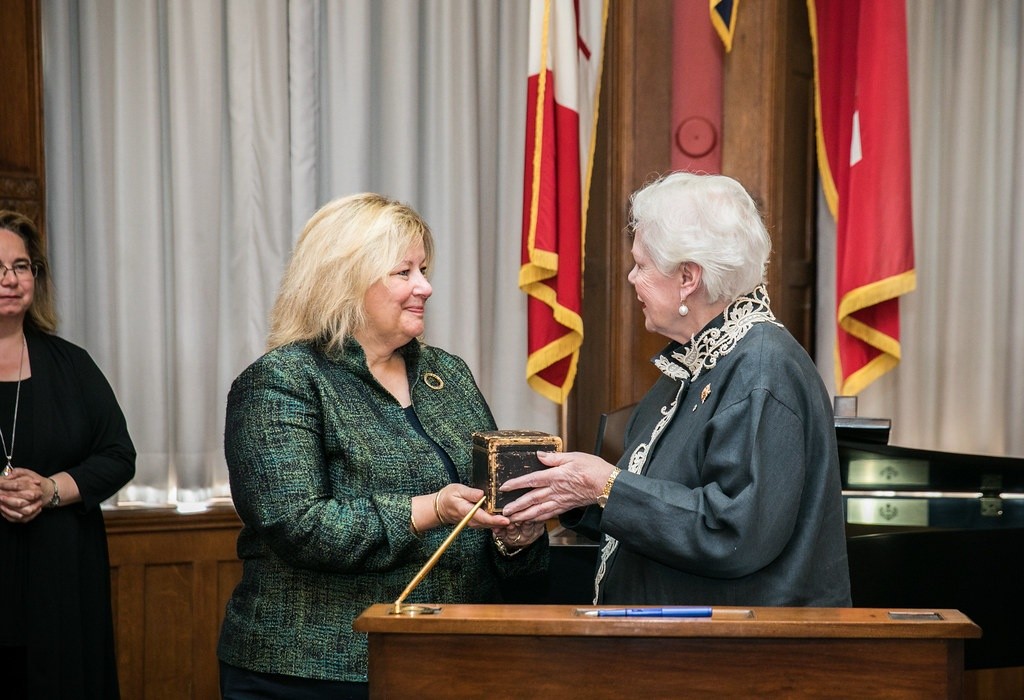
left=410, top=516, right=422, bottom=535
left=435, top=488, right=456, bottom=529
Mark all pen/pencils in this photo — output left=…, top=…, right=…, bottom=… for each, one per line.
left=584, top=607, right=712, bottom=618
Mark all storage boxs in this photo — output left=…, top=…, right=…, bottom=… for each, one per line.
left=469, top=430, right=564, bottom=515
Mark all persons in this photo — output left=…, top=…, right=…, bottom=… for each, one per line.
left=218, top=196, right=550, bottom=700
left=498, top=175, right=852, bottom=607
left=0, top=210, right=138, bottom=700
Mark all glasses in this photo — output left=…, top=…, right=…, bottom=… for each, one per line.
left=0, top=260, right=38, bottom=284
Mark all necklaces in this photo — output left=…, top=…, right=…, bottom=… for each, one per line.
left=0, top=334, right=26, bottom=475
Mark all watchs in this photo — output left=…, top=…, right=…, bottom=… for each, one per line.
left=49, top=478, right=60, bottom=507
left=597, top=468, right=621, bottom=508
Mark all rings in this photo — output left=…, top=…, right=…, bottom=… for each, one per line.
left=508, top=532, right=521, bottom=542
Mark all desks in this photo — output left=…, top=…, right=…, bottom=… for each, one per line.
left=351, top=604, right=980, bottom=699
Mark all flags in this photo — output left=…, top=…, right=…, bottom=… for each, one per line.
left=806, top=1, right=914, bottom=395
left=517, top=0, right=607, bottom=405
